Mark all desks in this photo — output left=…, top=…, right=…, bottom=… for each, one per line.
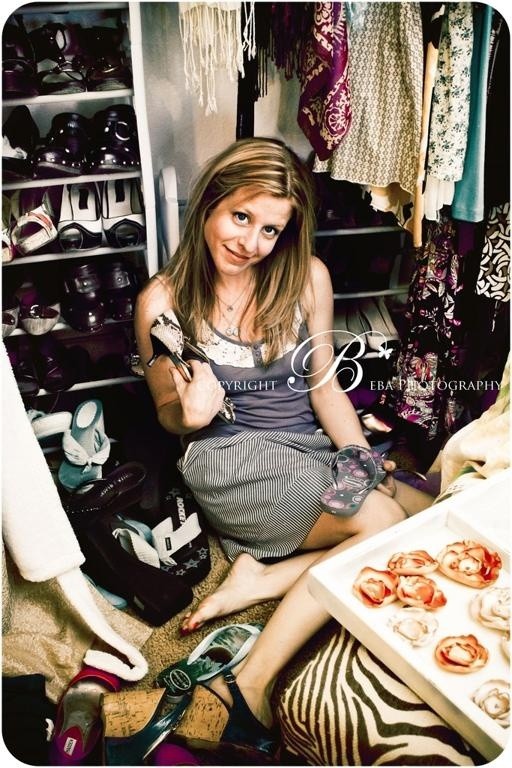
left=308, top=460, right=510, bottom=760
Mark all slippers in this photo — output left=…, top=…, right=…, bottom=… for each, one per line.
left=319, top=444, right=387, bottom=516
left=27, top=400, right=149, bottom=519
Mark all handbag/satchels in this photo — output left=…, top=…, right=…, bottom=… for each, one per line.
left=83, top=476, right=212, bottom=627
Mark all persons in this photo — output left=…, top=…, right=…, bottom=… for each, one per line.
left=132, top=134, right=408, bottom=731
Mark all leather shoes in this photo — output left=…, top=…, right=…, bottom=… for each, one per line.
left=333, top=301, right=368, bottom=360
left=2, top=15, right=145, bottom=396
left=360, top=296, right=400, bottom=353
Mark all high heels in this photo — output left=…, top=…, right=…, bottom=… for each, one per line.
left=147, top=309, right=237, bottom=426
left=52, top=622, right=277, bottom=764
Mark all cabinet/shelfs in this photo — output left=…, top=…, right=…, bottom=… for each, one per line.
left=4, top=2, right=163, bottom=398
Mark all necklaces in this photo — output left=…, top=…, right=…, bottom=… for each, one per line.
left=203, top=266, right=255, bottom=312
left=213, top=286, right=253, bottom=338
